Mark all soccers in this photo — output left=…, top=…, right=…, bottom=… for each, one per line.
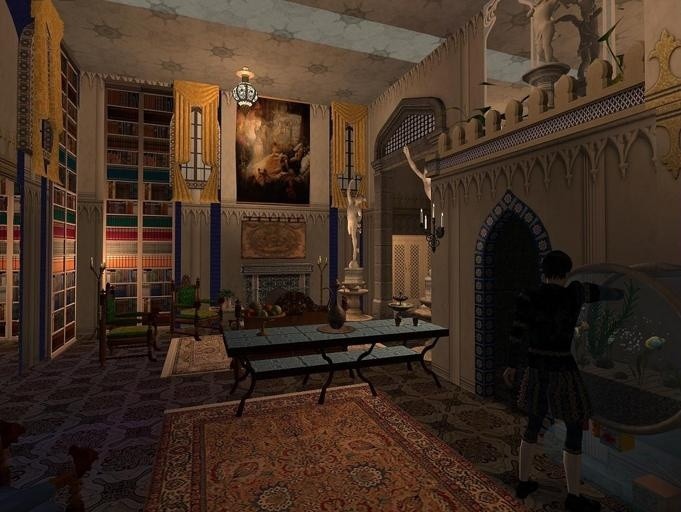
left=244, top=302, right=282, bottom=318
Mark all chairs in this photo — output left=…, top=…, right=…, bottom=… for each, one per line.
left=516, top=480, right=540, bottom=499
left=564, top=492, right=602, bottom=512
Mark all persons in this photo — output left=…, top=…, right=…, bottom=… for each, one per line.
left=509, top=249, right=602, bottom=511
left=525, top=0, right=559, bottom=66
left=348, top=178, right=364, bottom=261
